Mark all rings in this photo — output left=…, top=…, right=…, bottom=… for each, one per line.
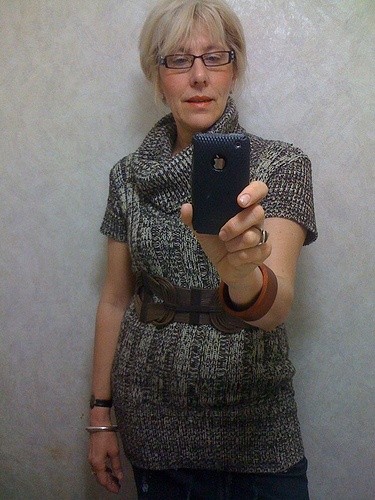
left=252, top=225, right=270, bottom=246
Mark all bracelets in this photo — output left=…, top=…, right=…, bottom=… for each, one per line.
left=217, top=263, right=278, bottom=322
left=85, top=425, right=119, bottom=433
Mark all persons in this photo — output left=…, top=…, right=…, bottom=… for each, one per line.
left=83, top=0, right=319, bottom=500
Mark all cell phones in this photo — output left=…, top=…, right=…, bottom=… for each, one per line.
left=190, top=133, right=251, bottom=234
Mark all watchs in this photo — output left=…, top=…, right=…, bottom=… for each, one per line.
left=89, top=395, right=112, bottom=409
